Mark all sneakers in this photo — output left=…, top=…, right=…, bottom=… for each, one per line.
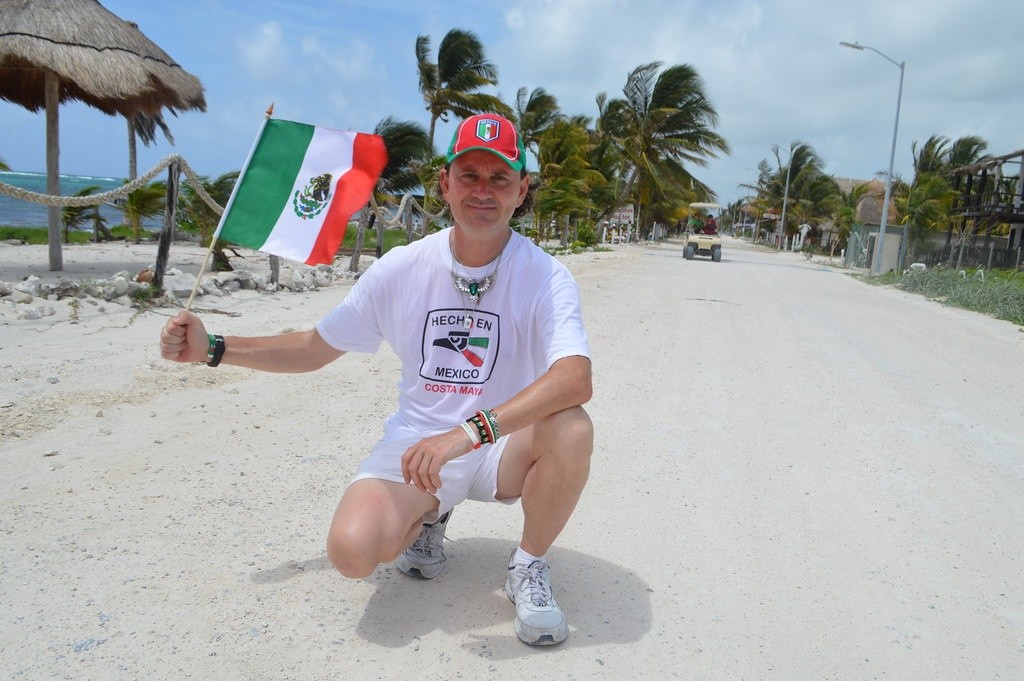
left=396, top=506, right=454, bottom=579
left=505, top=548, right=569, bottom=645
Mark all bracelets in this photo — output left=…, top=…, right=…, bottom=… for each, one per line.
left=201, top=334, right=226, bottom=368
left=462, top=408, right=500, bottom=450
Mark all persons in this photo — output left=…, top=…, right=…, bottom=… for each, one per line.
left=686, top=212, right=716, bottom=234
left=161, top=114, right=595, bottom=645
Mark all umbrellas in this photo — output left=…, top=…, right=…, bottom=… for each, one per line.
left=0, top=0, right=208, bottom=271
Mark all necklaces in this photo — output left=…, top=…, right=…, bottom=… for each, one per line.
left=449, top=233, right=510, bottom=330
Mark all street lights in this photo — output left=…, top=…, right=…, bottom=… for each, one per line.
left=837, top=39, right=906, bottom=279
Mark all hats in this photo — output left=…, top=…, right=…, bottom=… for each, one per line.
left=446, top=113, right=526, bottom=171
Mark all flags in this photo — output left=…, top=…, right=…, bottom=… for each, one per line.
left=219, top=119, right=389, bottom=267
